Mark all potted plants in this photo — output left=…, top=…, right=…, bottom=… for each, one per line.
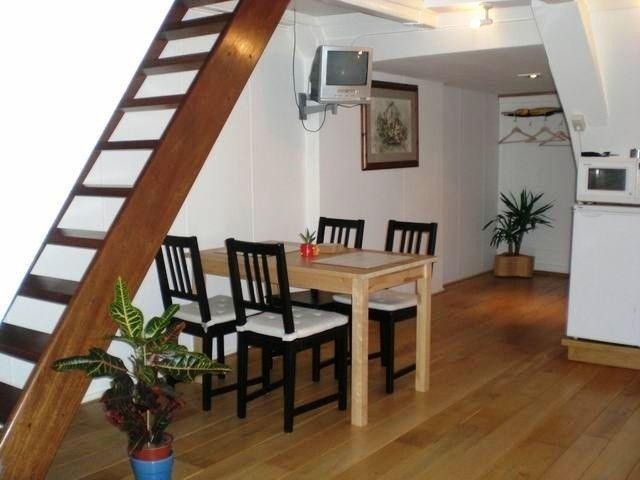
left=50, top=275, right=234, bottom=462
left=482, top=186, right=558, bottom=278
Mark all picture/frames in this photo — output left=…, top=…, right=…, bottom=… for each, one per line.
left=360, top=79, right=419, bottom=171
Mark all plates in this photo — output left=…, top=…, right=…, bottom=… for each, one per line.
left=317, top=243, right=344, bottom=254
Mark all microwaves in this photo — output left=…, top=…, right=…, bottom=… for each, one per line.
left=576, top=156, right=640, bottom=205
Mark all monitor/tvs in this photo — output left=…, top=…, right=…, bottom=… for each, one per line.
left=309, top=45, right=373, bottom=105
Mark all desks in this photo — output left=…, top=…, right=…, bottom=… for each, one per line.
left=194, top=241, right=438, bottom=428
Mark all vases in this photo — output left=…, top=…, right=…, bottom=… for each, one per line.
left=129, top=448, right=175, bottom=480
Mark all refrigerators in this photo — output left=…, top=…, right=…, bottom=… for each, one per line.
left=566, top=204, right=639, bottom=348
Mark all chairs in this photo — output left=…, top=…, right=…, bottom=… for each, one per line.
left=265, top=217, right=364, bottom=381
left=224, top=237, right=350, bottom=431
left=158, top=236, right=273, bottom=411
left=333, top=219, right=437, bottom=394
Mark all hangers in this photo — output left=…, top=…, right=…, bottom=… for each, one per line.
left=498, top=110, right=572, bottom=147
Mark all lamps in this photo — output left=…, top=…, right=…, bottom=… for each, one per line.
left=472, top=4, right=493, bottom=28
